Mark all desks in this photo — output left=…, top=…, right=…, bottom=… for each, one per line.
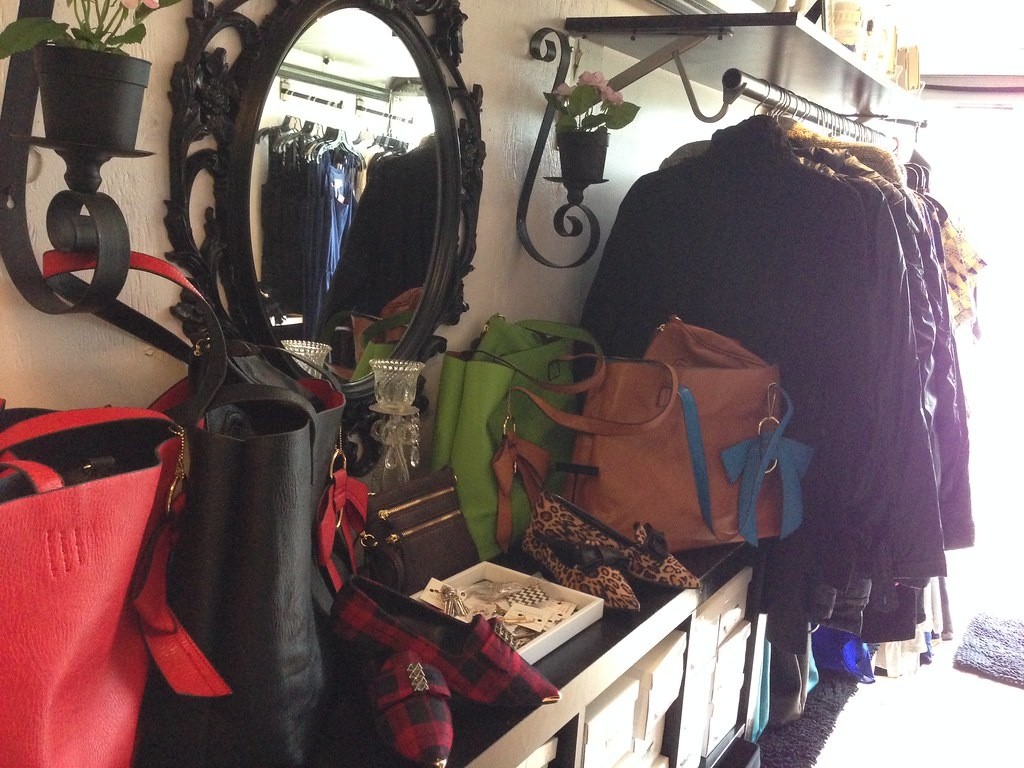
left=305, top=537, right=766, bottom=768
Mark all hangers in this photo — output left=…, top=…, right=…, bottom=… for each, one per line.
left=755, top=80, right=902, bottom=153
left=254, top=89, right=411, bottom=166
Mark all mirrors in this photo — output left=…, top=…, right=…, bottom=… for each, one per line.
left=165, top=1, right=488, bottom=479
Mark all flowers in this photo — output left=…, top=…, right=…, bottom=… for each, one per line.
left=0, top=0, right=182, bottom=57
left=542, top=70, right=641, bottom=133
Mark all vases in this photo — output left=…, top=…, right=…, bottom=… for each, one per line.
left=556, top=133, right=609, bottom=183
left=32, top=45, right=151, bottom=150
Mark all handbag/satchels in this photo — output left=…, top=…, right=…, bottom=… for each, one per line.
left=349, top=286, right=425, bottom=382
left=433, top=311, right=604, bottom=563
left=561, top=312, right=815, bottom=550
left=0, top=406, right=233, bottom=768
left=40, top=245, right=332, bottom=768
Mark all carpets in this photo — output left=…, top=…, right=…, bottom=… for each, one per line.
left=757, top=643, right=881, bottom=768
left=953, top=612, right=1024, bottom=690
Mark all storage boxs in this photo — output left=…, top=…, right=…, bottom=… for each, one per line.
left=409, top=561, right=604, bottom=665
left=516, top=569, right=762, bottom=768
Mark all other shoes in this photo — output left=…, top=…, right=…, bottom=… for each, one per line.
left=520, top=523, right=640, bottom=614
left=332, top=607, right=454, bottom=768
left=328, top=573, right=562, bottom=707
left=532, top=491, right=704, bottom=590
